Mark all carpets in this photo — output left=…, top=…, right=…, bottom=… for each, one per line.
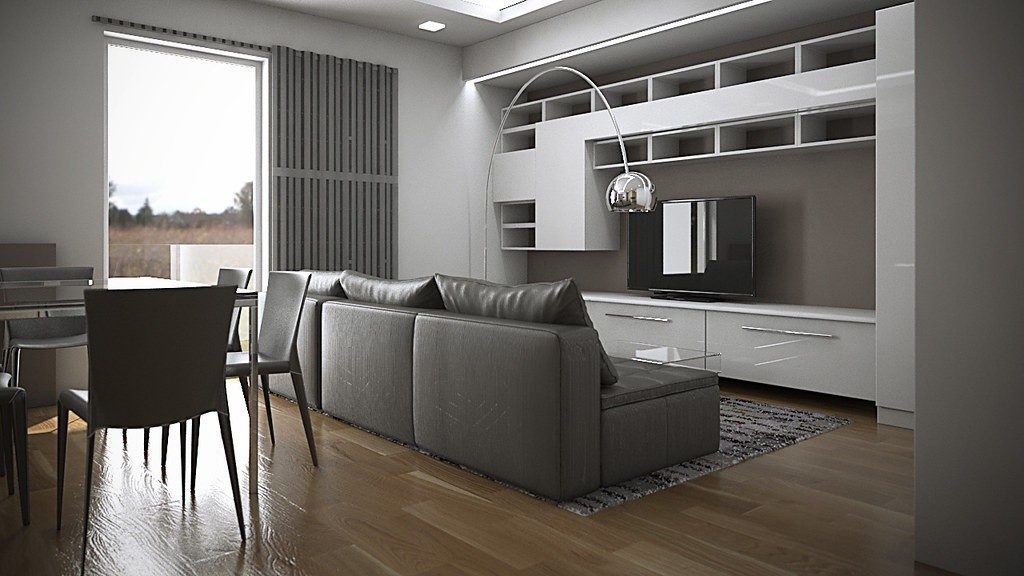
left=261, top=388, right=853, bottom=517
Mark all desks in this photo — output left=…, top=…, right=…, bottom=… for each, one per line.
left=0, top=277, right=259, bottom=523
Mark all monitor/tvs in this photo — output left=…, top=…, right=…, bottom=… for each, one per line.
left=626, top=195, right=757, bottom=303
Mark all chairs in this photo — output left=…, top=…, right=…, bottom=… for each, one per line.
left=0, top=267, right=318, bottom=576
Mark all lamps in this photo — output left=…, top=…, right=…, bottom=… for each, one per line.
left=484, top=66, right=656, bottom=281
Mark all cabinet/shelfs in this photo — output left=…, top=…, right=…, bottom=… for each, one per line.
left=582, top=292, right=876, bottom=401
left=492, top=0, right=876, bottom=251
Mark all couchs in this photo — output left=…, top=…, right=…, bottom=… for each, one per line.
left=265, top=270, right=720, bottom=501
